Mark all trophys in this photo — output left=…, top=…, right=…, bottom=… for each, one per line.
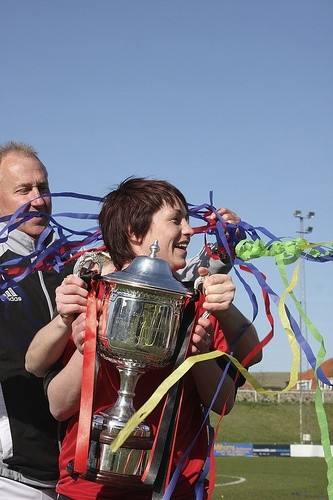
left=66, top=237, right=212, bottom=488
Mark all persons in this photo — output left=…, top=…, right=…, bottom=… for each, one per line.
left=24, top=250, right=264, bottom=500
left=45, top=176, right=244, bottom=500
left=0, top=140, right=245, bottom=500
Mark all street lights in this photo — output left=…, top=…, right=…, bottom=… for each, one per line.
left=293, top=210, right=314, bottom=390
